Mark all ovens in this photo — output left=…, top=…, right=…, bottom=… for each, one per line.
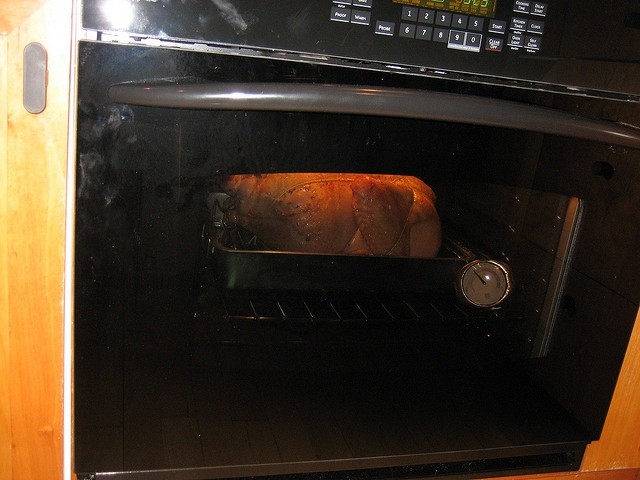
left=73, top=38, right=640, bottom=474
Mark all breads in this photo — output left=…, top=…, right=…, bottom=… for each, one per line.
left=219, top=172, right=444, bottom=259
